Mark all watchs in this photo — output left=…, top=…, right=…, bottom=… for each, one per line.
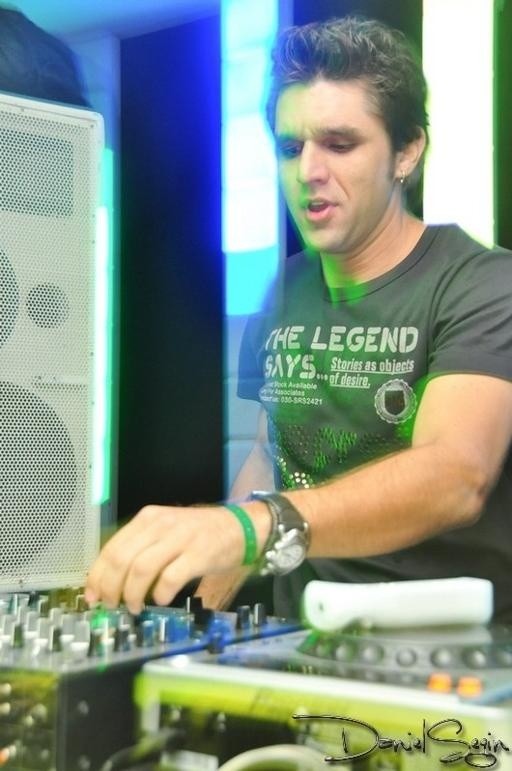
left=241, top=488, right=312, bottom=581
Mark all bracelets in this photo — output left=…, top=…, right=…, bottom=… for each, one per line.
left=219, top=501, right=259, bottom=569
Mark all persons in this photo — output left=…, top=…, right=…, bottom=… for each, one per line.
left=79, top=10, right=511, bottom=621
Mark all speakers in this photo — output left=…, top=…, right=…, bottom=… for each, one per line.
left=0, top=92, right=105, bottom=594
